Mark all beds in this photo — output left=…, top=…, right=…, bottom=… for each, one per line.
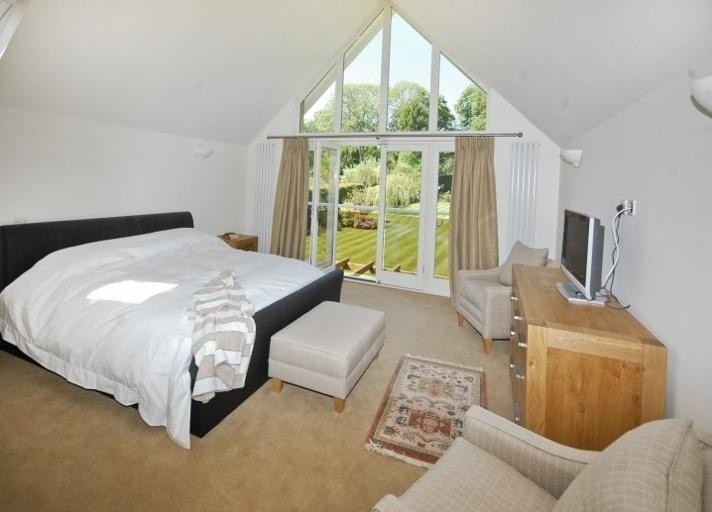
left=0, top=212, right=343, bottom=448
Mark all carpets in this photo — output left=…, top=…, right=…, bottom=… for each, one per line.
left=364, top=353, right=488, bottom=470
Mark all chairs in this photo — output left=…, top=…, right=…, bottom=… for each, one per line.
left=455, top=259, right=561, bottom=356
left=371, top=405, right=712, bottom=512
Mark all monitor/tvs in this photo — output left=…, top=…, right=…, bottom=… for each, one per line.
left=556, top=208, right=610, bottom=306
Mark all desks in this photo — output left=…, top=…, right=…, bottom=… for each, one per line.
left=509, top=263, right=668, bottom=451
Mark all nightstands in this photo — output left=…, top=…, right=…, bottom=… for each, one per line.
left=217, top=232, right=258, bottom=253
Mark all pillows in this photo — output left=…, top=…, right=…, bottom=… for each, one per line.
left=555, top=418, right=705, bottom=512
left=499, top=240, right=549, bottom=286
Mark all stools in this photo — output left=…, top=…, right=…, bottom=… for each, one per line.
left=267, top=301, right=386, bottom=413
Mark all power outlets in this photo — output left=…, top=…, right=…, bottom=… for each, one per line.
left=617, top=200, right=627, bottom=216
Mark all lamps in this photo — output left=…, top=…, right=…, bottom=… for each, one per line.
left=690, top=75, right=712, bottom=113
left=560, top=149, right=584, bottom=168
